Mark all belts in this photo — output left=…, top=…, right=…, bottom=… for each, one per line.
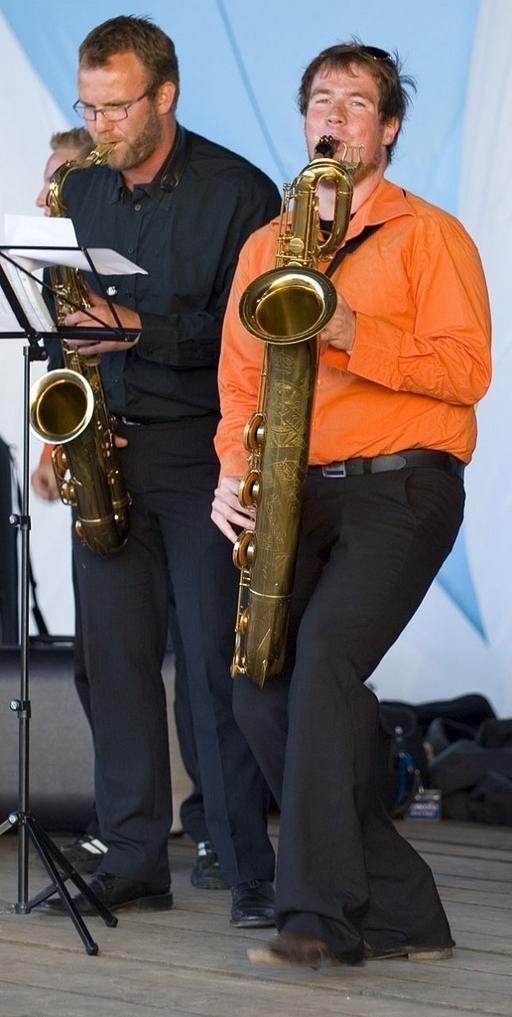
left=321, top=449, right=457, bottom=477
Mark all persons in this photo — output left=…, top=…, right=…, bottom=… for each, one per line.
left=39, top=15, right=287, bottom=926
left=210, top=44, right=493, bottom=971
left=29, top=129, right=231, bottom=889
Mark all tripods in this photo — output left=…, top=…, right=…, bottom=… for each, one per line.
left=1, top=356, right=118, bottom=955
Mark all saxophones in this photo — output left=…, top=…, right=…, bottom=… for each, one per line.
left=28, top=143, right=133, bottom=555
left=230, top=135, right=362, bottom=687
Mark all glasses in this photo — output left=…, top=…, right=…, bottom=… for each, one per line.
left=72, top=94, right=147, bottom=121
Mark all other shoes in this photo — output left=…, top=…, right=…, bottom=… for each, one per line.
left=248, top=929, right=363, bottom=968
left=360, top=935, right=453, bottom=960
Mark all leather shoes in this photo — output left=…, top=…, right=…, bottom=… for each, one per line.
left=230, top=882, right=276, bottom=929
left=45, top=874, right=173, bottom=911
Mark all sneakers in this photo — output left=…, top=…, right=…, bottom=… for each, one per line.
left=191, top=841, right=232, bottom=889
